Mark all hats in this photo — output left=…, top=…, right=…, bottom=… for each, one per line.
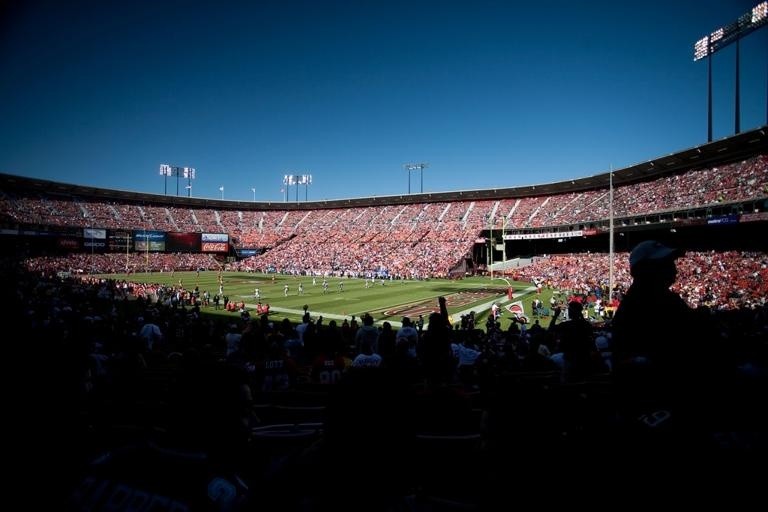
left=629, top=241, right=686, bottom=274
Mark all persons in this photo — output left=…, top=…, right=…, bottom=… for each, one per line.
left=0, top=153, right=768, bottom=511
